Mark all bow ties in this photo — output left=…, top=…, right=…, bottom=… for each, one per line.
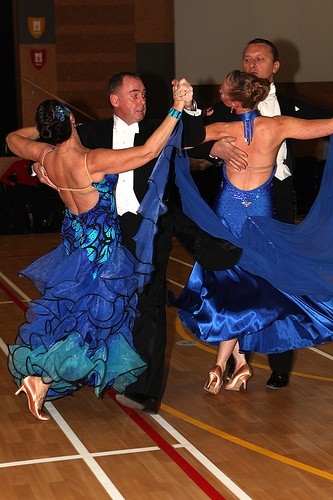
left=113, top=115, right=139, bottom=136
left=269, top=83, right=276, bottom=97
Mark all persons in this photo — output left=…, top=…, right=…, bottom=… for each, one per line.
left=7, top=72, right=184, bottom=421
left=0, top=158, right=46, bottom=236
left=176, top=70, right=333, bottom=394
left=30, top=73, right=207, bottom=414
left=185, top=38, right=333, bottom=389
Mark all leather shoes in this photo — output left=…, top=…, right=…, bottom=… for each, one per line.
left=116, top=388, right=159, bottom=415
left=266, top=374, right=289, bottom=389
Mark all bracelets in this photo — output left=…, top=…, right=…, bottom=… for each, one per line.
left=167, top=107, right=182, bottom=120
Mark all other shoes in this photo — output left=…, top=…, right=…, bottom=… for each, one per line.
left=225, top=353, right=251, bottom=380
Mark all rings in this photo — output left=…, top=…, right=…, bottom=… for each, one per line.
left=184, top=90, right=186, bottom=96
left=185, top=91, right=186, bottom=95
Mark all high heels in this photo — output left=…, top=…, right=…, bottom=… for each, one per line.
left=203, top=365, right=224, bottom=394
left=15, top=375, right=50, bottom=421
left=225, top=365, right=252, bottom=391
